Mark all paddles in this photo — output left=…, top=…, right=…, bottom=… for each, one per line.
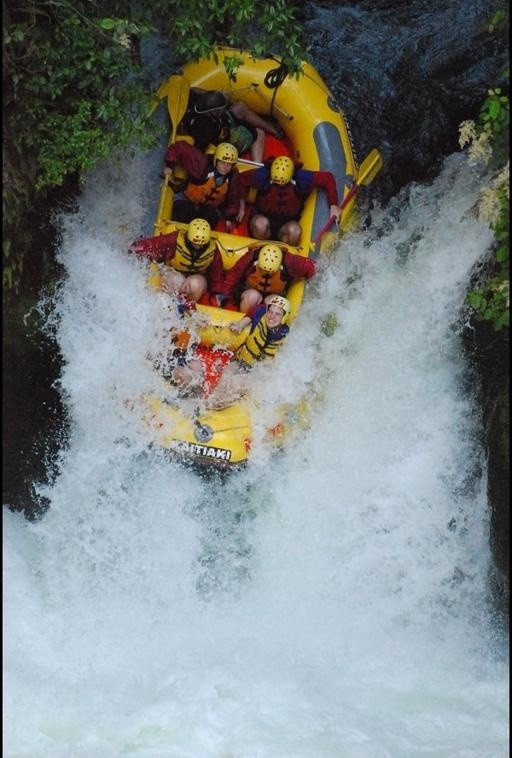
left=154, top=74, right=190, bottom=228
left=308, top=149, right=383, bottom=252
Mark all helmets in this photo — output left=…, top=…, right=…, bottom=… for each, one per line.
left=194, top=90, right=226, bottom=114
left=270, top=156, right=295, bottom=186
left=214, top=143, right=239, bottom=169
left=267, top=296, right=290, bottom=325
left=258, top=244, right=283, bottom=275
left=187, top=218, right=211, bottom=244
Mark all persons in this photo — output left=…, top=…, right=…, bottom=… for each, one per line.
left=127, top=218, right=226, bottom=307
left=220, top=154, right=342, bottom=246
left=182, top=86, right=286, bottom=164
left=162, top=140, right=246, bottom=234
left=151, top=291, right=207, bottom=384
left=216, top=243, right=317, bottom=315
left=207, top=293, right=291, bottom=409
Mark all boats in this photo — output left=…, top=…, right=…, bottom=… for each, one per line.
left=97, top=43, right=365, bottom=475
